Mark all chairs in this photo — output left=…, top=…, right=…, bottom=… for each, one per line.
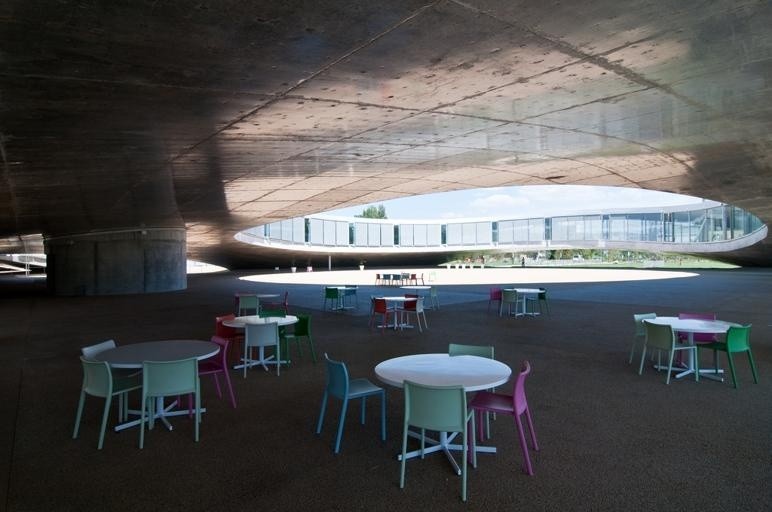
left=697, top=322, right=758, bottom=389
left=465, top=361, right=538, bottom=477
left=398, top=379, right=477, bottom=501
left=315, top=350, right=387, bottom=456
left=444, top=342, right=497, bottom=441
left=639, top=320, right=699, bottom=384
left=629, top=312, right=678, bottom=363
left=70, top=338, right=152, bottom=450
left=678, top=313, right=720, bottom=374
left=139, top=357, right=201, bottom=446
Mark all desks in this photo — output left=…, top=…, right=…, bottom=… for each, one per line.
left=96, top=340, right=221, bottom=431
left=651, top=315, right=743, bottom=381
left=373, top=355, right=512, bottom=475
